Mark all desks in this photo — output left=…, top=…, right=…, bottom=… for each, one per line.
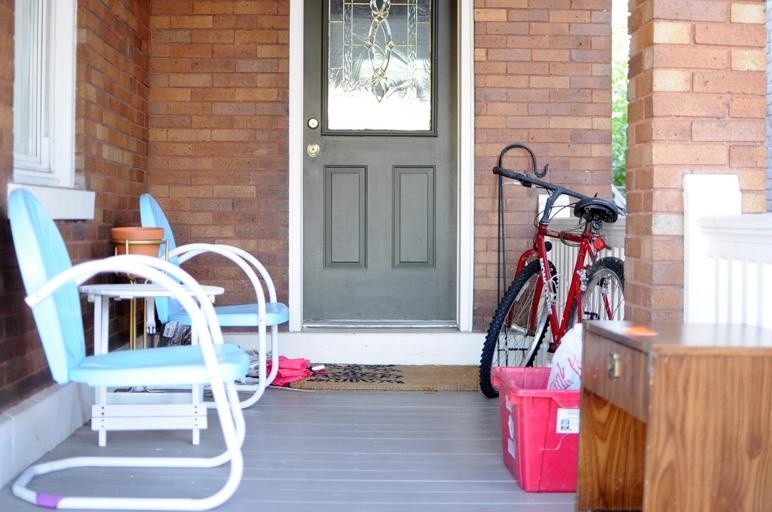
left=78, top=284, right=224, bottom=448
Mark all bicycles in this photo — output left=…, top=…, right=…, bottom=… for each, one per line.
left=475, top=161, right=626, bottom=402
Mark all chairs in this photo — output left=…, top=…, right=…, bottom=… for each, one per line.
left=7, top=188, right=249, bottom=511
left=140, top=194, right=290, bottom=409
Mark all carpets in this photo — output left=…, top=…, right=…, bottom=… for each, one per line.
left=289, top=363, right=480, bottom=391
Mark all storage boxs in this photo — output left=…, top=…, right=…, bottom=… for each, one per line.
left=491, top=366, right=580, bottom=493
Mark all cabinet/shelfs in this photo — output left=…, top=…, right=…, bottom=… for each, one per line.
left=576, top=319, right=772, bottom=512
